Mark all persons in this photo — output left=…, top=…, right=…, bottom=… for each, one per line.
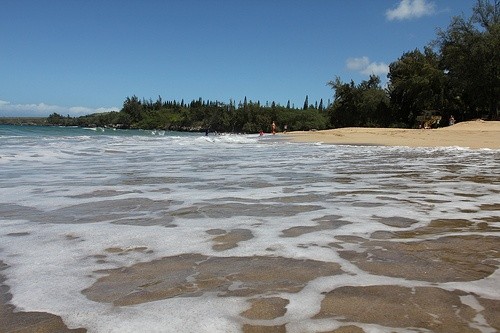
left=271, top=121, right=276, bottom=134
left=205, top=129, right=209, bottom=136
left=283, top=123, right=288, bottom=135
left=259, top=130, right=263, bottom=135
left=449, top=115, right=455, bottom=125
